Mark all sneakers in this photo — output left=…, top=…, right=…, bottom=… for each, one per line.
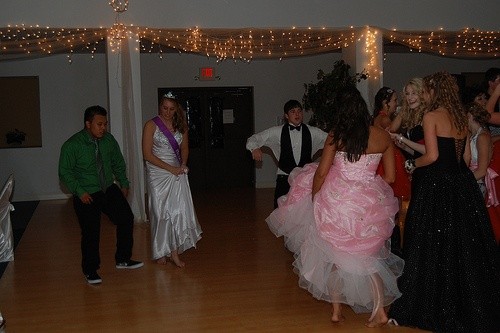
left=115, top=260, right=145, bottom=270
left=81, top=271, right=103, bottom=284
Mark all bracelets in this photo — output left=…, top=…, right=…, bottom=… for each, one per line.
left=399, top=136, right=404, bottom=142
left=404, top=160, right=417, bottom=174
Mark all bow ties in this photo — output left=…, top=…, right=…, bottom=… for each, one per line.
left=288, top=124, right=302, bottom=131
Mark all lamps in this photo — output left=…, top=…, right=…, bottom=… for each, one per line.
left=192, top=67, right=221, bottom=81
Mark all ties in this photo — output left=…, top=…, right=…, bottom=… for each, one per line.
left=89, top=138, right=107, bottom=195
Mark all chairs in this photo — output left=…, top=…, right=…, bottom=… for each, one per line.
left=0, top=173, right=15, bottom=262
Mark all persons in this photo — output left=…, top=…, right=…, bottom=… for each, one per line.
left=247, top=66, right=500, bottom=333
left=58, top=105, right=145, bottom=283
left=143, top=92, right=203, bottom=268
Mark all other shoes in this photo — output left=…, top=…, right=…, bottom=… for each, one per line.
left=169, top=255, right=185, bottom=269
left=157, top=257, right=167, bottom=265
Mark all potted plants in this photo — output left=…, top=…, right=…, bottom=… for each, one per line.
left=5, top=128, right=29, bottom=147
